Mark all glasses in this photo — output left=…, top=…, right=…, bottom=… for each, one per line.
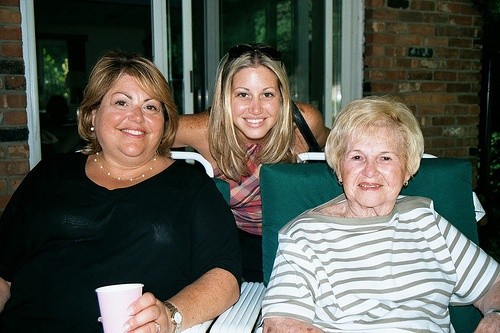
left=229, top=45, right=283, bottom=70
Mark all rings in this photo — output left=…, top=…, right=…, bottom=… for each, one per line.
left=155, top=322, right=160, bottom=333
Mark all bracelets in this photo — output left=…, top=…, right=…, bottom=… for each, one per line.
left=487, top=308, right=500, bottom=314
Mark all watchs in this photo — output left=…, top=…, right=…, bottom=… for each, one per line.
left=163, top=301, right=183, bottom=333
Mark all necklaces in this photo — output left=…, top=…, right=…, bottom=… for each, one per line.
left=94, top=152, right=156, bottom=181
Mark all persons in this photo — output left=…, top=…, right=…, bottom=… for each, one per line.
left=256, top=99, right=500, bottom=333
left=0, top=52, right=242, bottom=333
left=172, top=45, right=331, bottom=282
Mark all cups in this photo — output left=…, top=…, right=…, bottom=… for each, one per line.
left=95, top=283, right=145, bottom=333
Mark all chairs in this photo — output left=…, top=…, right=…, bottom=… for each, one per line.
left=166, top=151, right=483, bottom=333
